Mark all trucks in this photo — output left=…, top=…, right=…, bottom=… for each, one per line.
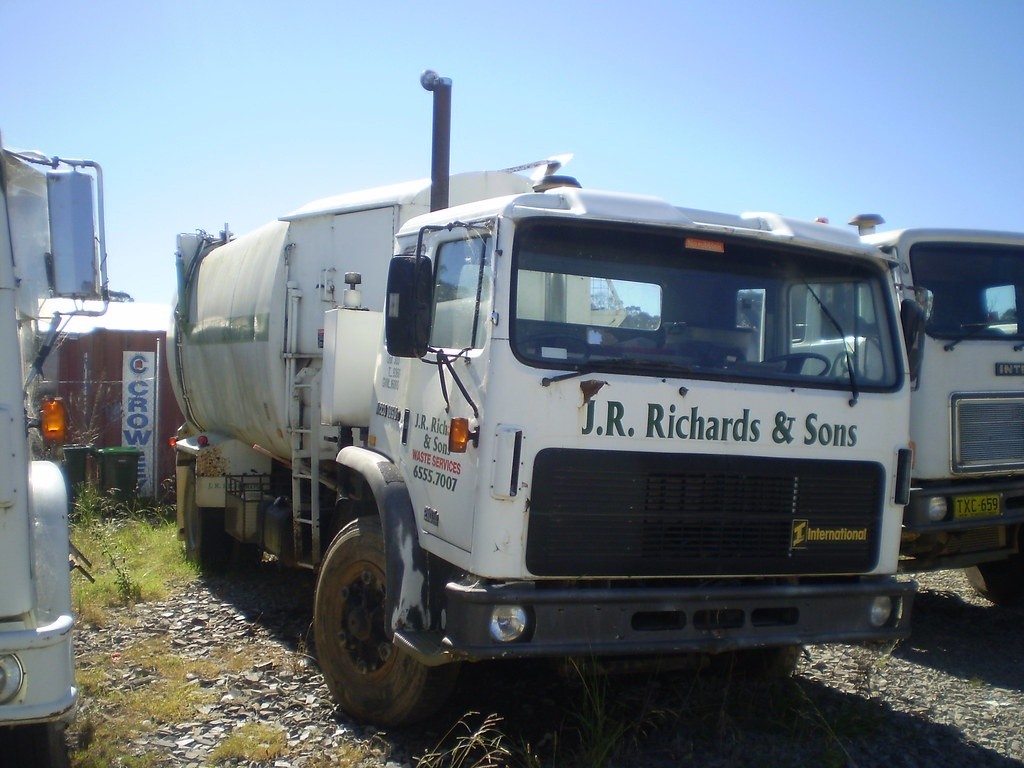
left=813, top=210, right=1024, bottom=615
left=164, top=68, right=929, bottom=741
left=0, top=131, right=136, bottom=768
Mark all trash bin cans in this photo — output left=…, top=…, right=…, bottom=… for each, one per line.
left=90, top=443, right=145, bottom=520
left=57, top=443, right=92, bottom=524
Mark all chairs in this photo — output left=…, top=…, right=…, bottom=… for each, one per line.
left=942, top=292, right=990, bottom=332
left=663, top=324, right=757, bottom=358
